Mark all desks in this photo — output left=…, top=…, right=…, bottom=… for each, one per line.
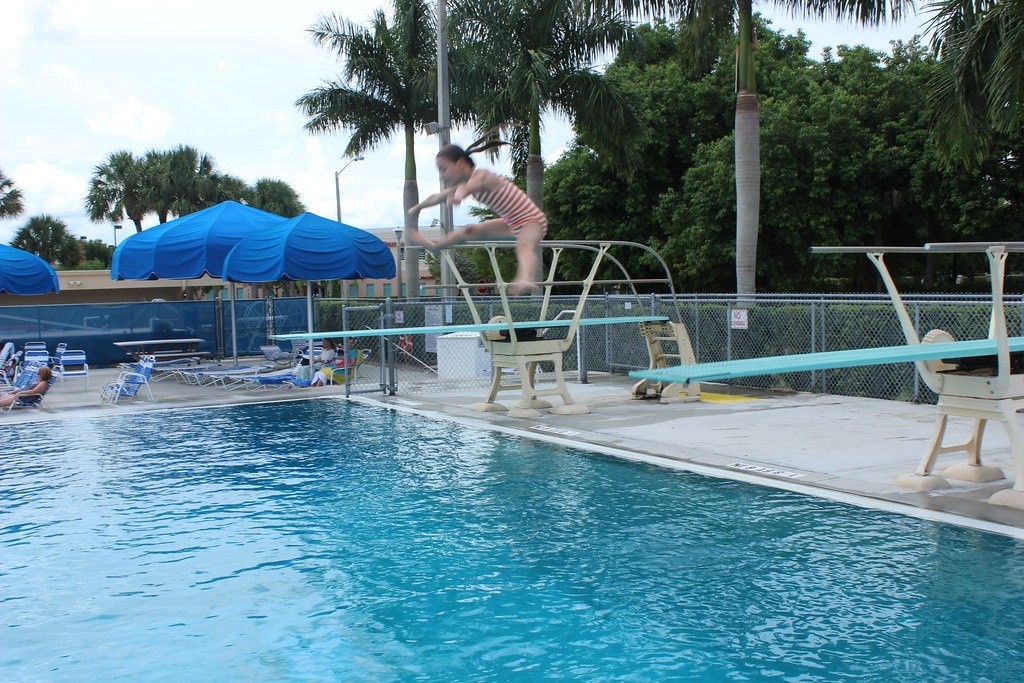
left=436, top=332, right=485, bottom=380
left=111, top=338, right=206, bottom=355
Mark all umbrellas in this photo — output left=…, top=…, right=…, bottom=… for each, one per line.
left=110, top=201, right=395, bottom=370
left=0, top=244, right=61, bottom=295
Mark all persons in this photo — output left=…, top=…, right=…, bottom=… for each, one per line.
left=0, top=358, right=51, bottom=407
left=403, top=144, right=548, bottom=294
left=311, top=336, right=356, bottom=387
left=296, top=338, right=336, bottom=388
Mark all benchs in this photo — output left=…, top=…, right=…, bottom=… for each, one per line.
left=127, top=349, right=214, bottom=366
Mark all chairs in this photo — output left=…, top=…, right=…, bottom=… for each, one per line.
left=115, top=363, right=296, bottom=391
left=0, top=342, right=90, bottom=416
left=259, top=330, right=372, bottom=388
left=98, top=355, right=156, bottom=405
